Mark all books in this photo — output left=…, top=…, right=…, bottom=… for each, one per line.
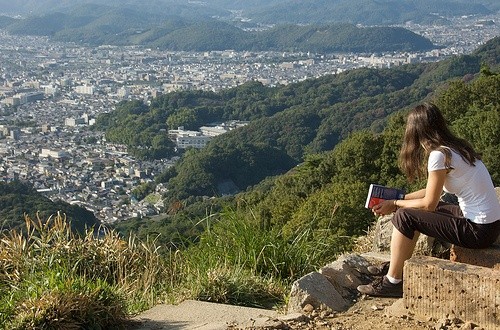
left=364, top=183, right=406, bottom=212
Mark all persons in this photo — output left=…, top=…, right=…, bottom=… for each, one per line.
left=357, top=103, right=500, bottom=298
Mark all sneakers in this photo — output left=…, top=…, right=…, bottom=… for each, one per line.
left=367, top=261, right=390, bottom=276
left=357, top=275, right=403, bottom=298
